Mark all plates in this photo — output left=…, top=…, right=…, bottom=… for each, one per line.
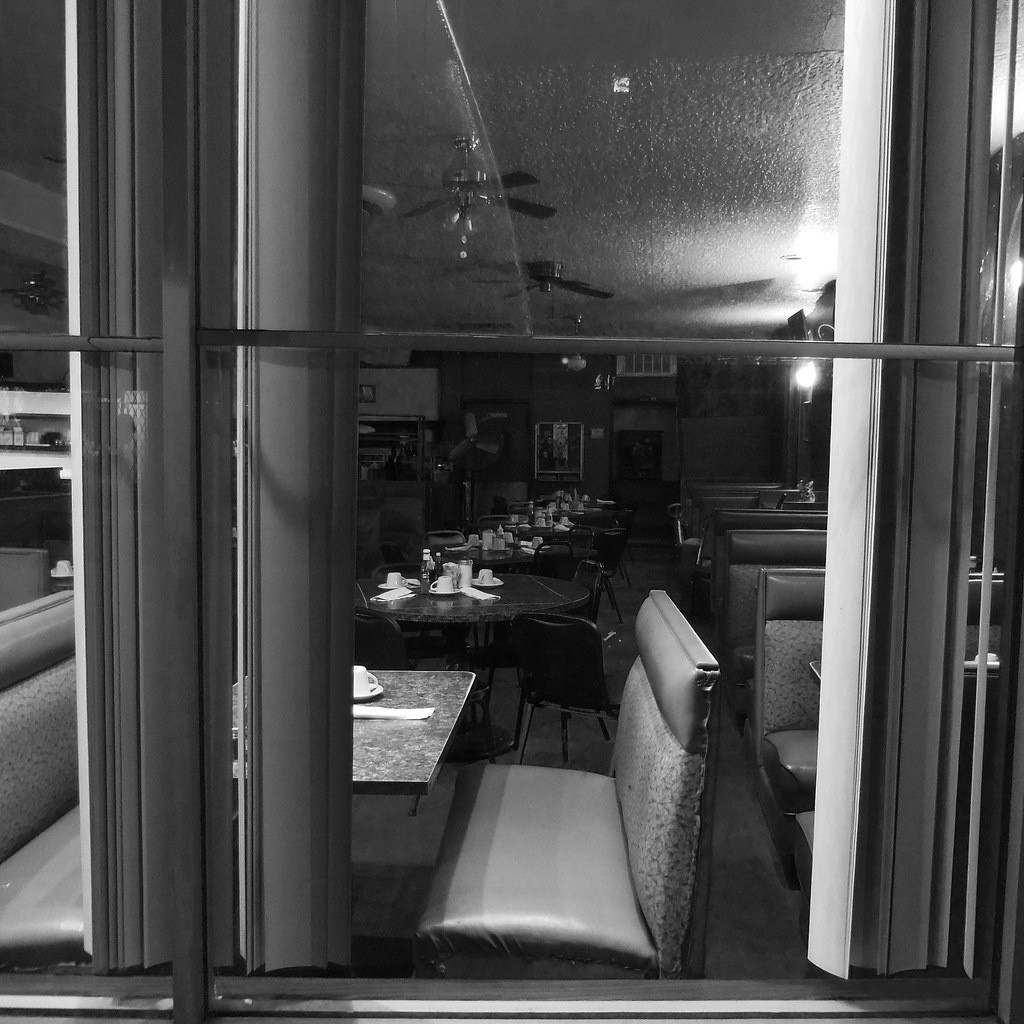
left=353, top=683, right=384, bottom=700
left=51, top=570, right=74, bottom=577
left=473, top=579, right=504, bottom=586
left=378, top=582, right=407, bottom=589
left=429, top=588, right=460, bottom=594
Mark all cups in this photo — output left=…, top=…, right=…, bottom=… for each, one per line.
left=458, top=560, right=473, bottom=589
left=469, top=534, right=479, bottom=548
left=431, top=576, right=454, bottom=594
left=57, top=560, right=72, bottom=575
left=480, top=568, right=494, bottom=584
left=504, top=532, right=513, bottom=544
left=510, top=514, right=519, bottom=523
left=560, top=516, right=568, bottom=526
left=496, top=539, right=505, bottom=550
left=353, top=664, right=378, bottom=696
left=536, top=518, right=546, bottom=529
left=387, top=572, right=408, bottom=589
left=532, top=537, right=544, bottom=549
left=483, top=529, right=495, bottom=552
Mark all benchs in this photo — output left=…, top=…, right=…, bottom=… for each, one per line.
left=751, top=565, right=1004, bottom=889
left=0, top=547, right=52, bottom=611
left=788, top=811, right=815, bottom=895
left=41, top=510, right=73, bottom=567
left=680, top=478, right=835, bottom=762
left=410, top=589, right=722, bottom=978
left=0, top=590, right=83, bottom=971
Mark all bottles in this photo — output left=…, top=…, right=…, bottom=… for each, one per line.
left=527, top=500, right=534, bottom=525
left=420, top=546, right=442, bottom=593
left=546, top=506, right=553, bottom=527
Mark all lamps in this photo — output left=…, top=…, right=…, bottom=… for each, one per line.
left=437, top=189, right=476, bottom=232
left=567, top=353, right=586, bottom=372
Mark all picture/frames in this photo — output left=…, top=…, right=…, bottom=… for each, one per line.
left=358, top=415, right=422, bottom=485
left=535, top=421, right=584, bottom=474
left=616, top=429, right=663, bottom=485
left=359, top=384, right=376, bottom=403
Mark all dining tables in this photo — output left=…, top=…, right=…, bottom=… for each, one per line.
left=807, top=653, right=1000, bottom=704
left=464, top=520, right=608, bottom=541
left=509, top=505, right=623, bottom=522
left=354, top=573, right=590, bottom=818
left=423, top=542, right=599, bottom=668
left=232, top=671, right=477, bottom=798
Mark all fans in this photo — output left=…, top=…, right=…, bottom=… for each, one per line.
left=470, top=261, right=615, bottom=299
left=441, top=407, right=504, bottom=532
left=392, top=131, right=557, bottom=220
left=0, top=274, right=66, bottom=307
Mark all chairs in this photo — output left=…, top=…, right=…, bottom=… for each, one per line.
left=354, top=605, right=496, bottom=763
left=511, top=610, right=621, bottom=764
left=363, top=491, right=639, bottom=690
